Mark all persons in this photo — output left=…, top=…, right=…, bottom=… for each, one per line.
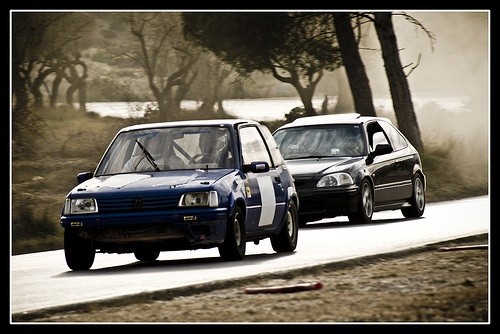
left=120, top=137, right=184, bottom=172
left=190, top=135, right=232, bottom=169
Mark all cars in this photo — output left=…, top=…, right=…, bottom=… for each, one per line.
left=58, top=118, right=304, bottom=271
left=272, top=111, right=426, bottom=226
